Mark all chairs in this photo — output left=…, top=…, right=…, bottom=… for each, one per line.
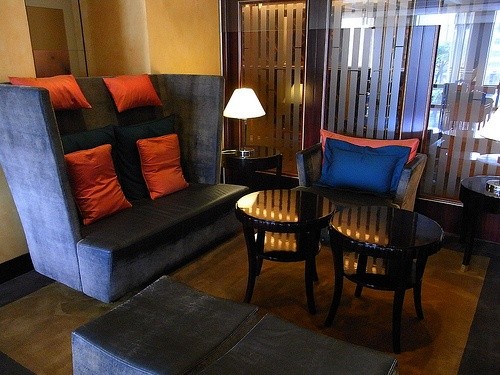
left=292, top=129, right=427, bottom=212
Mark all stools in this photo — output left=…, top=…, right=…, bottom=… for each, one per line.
left=196, top=313, right=398, bottom=375
left=72, top=275, right=259, bottom=375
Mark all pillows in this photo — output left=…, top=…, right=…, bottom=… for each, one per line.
left=319, top=137, right=411, bottom=202
left=8, top=75, right=92, bottom=111
left=102, top=74, right=163, bottom=112
left=64, top=144, right=133, bottom=225
left=136, top=133, right=189, bottom=200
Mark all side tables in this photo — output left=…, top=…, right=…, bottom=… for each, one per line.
left=220, top=145, right=283, bottom=193
left=459, top=175, right=500, bottom=273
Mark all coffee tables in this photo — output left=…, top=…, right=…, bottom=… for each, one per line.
left=235, top=189, right=337, bottom=316
left=324, top=206, right=444, bottom=354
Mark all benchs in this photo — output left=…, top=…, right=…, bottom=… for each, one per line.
left=0, top=74, right=248, bottom=304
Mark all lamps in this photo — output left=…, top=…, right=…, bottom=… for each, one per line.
left=223, top=88, right=266, bottom=155
